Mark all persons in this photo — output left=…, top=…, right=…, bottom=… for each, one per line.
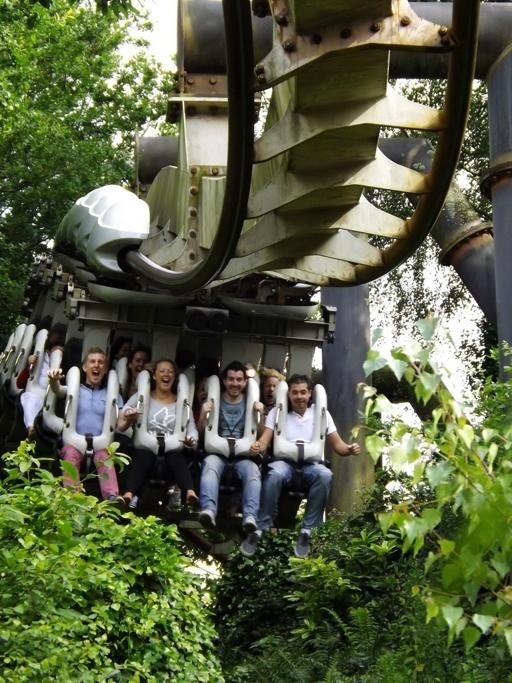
left=0, top=311, right=361, bottom=559
left=13, top=323, right=360, bottom=543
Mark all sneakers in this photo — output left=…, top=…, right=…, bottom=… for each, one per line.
left=188, top=493, right=201, bottom=511
left=115, top=495, right=137, bottom=509
left=198, top=510, right=217, bottom=530
left=295, top=529, right=311, bottom=557
left=241, top=516, right=258, bottom=531
left=240, top=532, right=262, bottom=556
